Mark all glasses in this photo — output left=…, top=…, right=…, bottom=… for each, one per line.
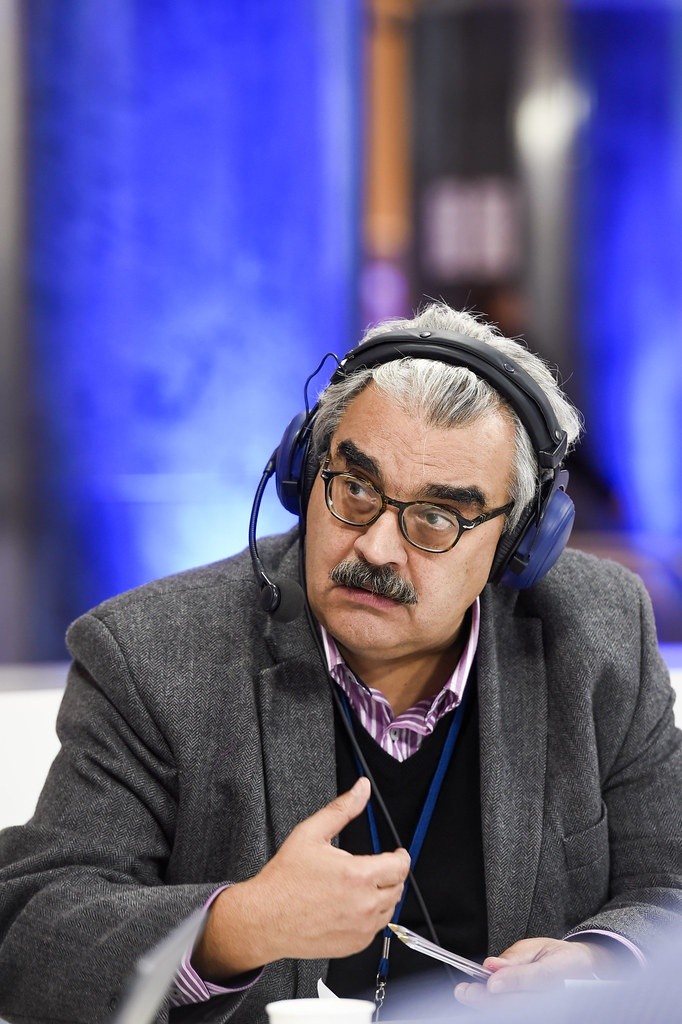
left=321, top=431, right=515, bottom=554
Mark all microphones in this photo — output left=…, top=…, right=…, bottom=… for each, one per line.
left=249, top=446, right=305, bottom=623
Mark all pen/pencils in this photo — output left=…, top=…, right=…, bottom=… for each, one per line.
left=387, top=922, right=495, bottom=984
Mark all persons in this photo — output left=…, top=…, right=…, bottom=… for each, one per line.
left=0, top=304, right=682, bottom=1024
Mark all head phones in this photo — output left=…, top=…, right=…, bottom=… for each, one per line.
left=275, top=328, right=576, bottom=594
